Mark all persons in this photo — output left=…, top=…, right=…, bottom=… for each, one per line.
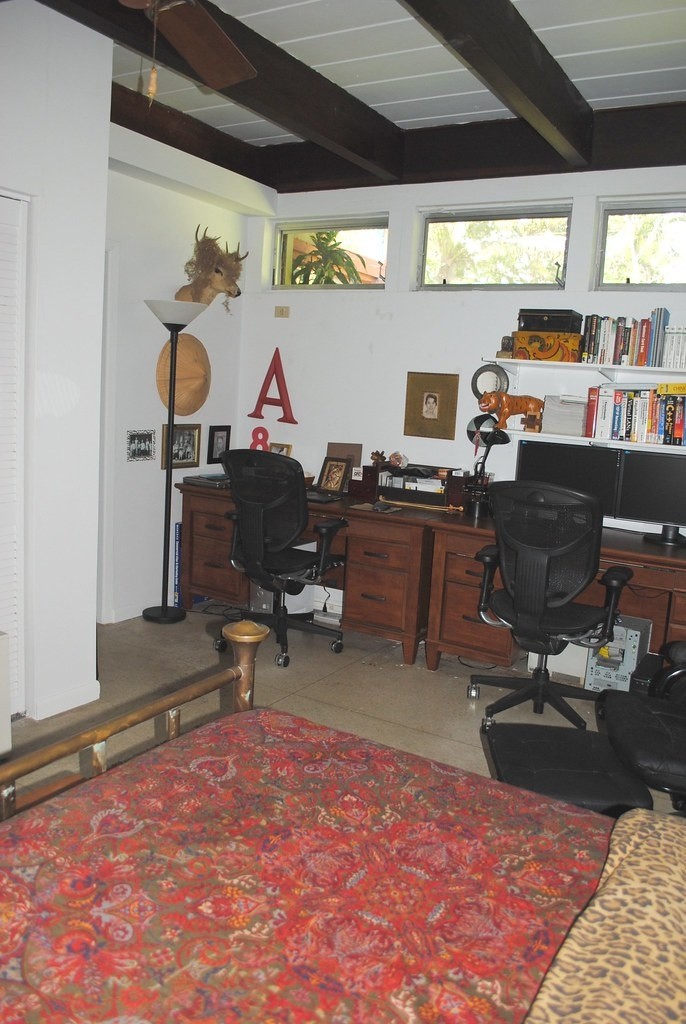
left=423, top=391, right=439, bottom=420
left=125, top=429, right=227, bottom=462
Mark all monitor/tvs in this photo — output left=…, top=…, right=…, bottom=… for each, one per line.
left=515, top=440, right=686, bottom=528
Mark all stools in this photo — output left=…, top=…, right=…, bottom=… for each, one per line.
left=487, top=722, right=655, bottom=822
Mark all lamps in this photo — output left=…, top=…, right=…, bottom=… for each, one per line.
left=141, top=299, right=210, bottom=624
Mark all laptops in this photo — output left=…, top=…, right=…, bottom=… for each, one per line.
left=183, top=475, right=231, bottom=489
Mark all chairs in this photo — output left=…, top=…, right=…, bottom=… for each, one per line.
left=604, top=639, right=686, bottom=810
left=212, top=449, right=350, bottom=669
left=467, top=480, right=634, bottom=737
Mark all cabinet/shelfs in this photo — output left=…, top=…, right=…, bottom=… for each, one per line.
left=473, top=352, right=686, bottom=452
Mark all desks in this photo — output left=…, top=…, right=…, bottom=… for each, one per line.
left=174, top=482, right=448, bottom=666
left=423, top=510, right=686, bottom=697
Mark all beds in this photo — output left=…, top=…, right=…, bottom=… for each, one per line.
left=0, top=618, right=686, bottom=1024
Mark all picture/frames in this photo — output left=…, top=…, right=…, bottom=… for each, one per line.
left=161, top=423, right=201, bottom=470
left=127, top=430, right=158, bottom=463
left=268, top=442, right=292, bottom=458
left=317, top=456, right=352, bottom=496
left=207, top=424, right=231, bottom=464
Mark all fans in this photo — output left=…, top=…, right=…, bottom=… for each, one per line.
left=117, top=1, right=259, bottom=107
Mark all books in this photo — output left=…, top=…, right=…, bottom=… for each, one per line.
left=583, top=308, right=686, bottom=447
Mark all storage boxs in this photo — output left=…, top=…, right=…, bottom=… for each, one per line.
left=511, top=308, right=585, bottom=362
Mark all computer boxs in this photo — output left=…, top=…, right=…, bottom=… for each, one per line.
left=629, top=653, right=663, bottom=696
